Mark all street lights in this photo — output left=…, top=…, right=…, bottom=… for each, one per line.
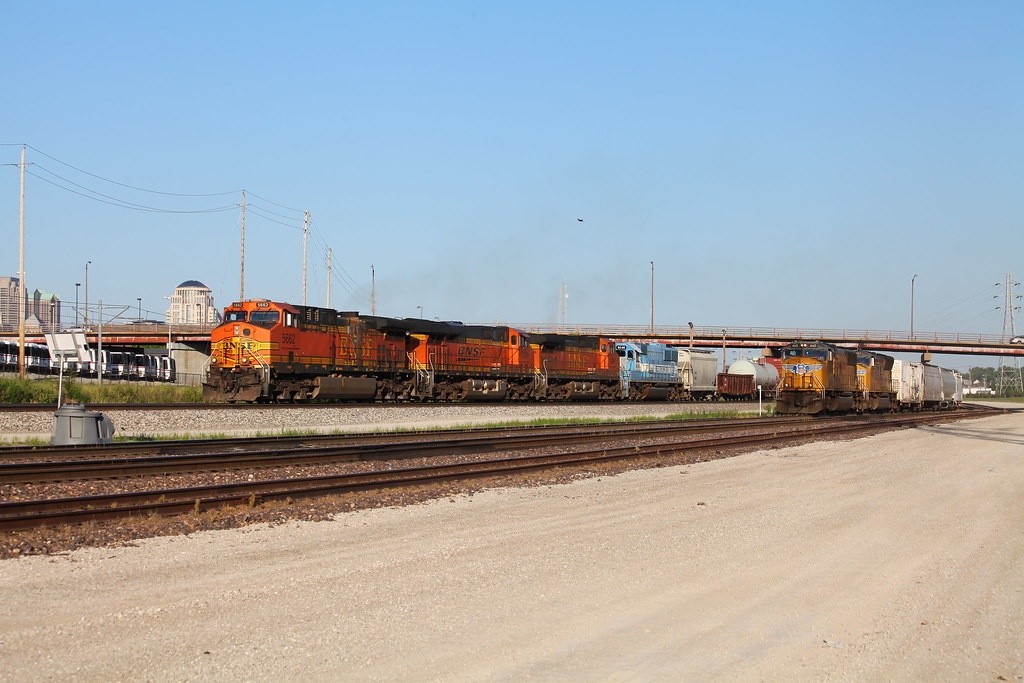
left=85, top=260, right=92, bottom=330
left=721, top=329, right=727, bottom=374
left=417, top=305, right=423, bottom=319
left=195, top=303, right=202, bottom=325
left=911, top=274, right=920, bottom=340
left=74, top=282, right=81, bottom=326
left=649, top=261, right=654, bottom=334
left=136, top=297, right=142, bottom=321
left=688, top=321, right=695, bottom=348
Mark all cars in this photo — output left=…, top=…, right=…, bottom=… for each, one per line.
left=1009, top=335, right=1024, bottom=344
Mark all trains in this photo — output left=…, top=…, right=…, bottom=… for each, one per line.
left=201, top=295, right=782, bottom=406
left=766, top=336, right=963, bottom=417
left=0, top=339, right=178, bottom=383
